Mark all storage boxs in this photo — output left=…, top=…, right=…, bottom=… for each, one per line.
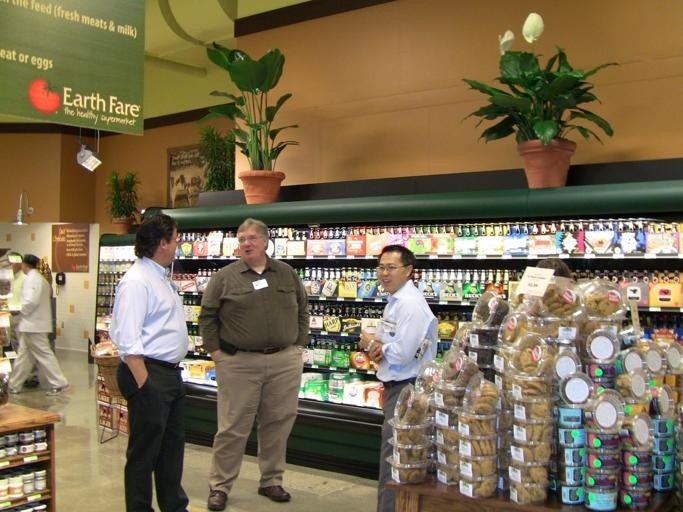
left=95, top=329, right=129, bottom=436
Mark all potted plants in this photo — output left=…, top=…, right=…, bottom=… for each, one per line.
left=200, top=36, right=304, bottom=207
left=104, top=169, right=144, bottom=235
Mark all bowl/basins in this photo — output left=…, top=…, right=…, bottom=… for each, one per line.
left=386, top=331, right=683, bottom=512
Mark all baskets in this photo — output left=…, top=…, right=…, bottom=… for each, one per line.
left=91, top=340, right=125, bottom=397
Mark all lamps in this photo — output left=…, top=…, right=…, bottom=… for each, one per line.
left=9, top=188, right=33, bottom=228
left=73, top=126, right=103, bottom=173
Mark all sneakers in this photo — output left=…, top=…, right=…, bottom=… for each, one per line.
left=259, top=483, right=291, bottom=502
left=46, top=382, right=70, bottom=396
left=207, top=486, right=228, bottom=511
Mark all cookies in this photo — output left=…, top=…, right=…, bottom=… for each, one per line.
left=394, top=284, right=631, bottom=505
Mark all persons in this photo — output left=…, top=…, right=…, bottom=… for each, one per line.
left=198, top=218, right=309, bottom=511
left=360, top=245, right=438, bottom=512
left=108, top=215, right=190, bottom=512
left=7, top=255, right=70, bottom=397
left=536, top=258, right=571, bottom=278
left=6, top=252, right=39, bottom=388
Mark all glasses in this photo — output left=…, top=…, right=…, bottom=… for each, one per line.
left=376, top=263, right=411, bottom=274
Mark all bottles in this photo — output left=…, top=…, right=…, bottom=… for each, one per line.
left=374, top=219, right=683, bottom=359
left=0, top=427, right=48, bottom=512
left=98, top=264, right=132, bottom=315
left=172, top=231, right=242, bottom=358
left=266, top=225, right=372, bottom=375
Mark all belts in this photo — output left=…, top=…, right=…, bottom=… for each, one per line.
left=144, top=356, right=185, bottom=370
left=235, top=346, right=290, bottom=354
left=382, top=377, right=417, bottom=387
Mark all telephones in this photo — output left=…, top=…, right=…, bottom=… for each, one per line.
left=55, top=273, right=65, bottom=284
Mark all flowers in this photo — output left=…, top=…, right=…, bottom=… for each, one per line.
left=458, top=12, right=619, bottom=146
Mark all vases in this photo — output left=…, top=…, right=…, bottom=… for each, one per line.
left=514, top=139, right=577, bottom=190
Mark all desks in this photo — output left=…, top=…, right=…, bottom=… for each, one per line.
left=380, top=481, right=682, bottom=512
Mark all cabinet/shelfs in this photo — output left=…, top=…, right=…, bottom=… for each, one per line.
left=91, top=234, right=140, bottom=364
left=143, top=178, right=682, bottom=478
left=0, top=401, right=63, bottom=512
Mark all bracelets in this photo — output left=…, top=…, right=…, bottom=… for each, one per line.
left=364, top=339, right=374, bottom=351
